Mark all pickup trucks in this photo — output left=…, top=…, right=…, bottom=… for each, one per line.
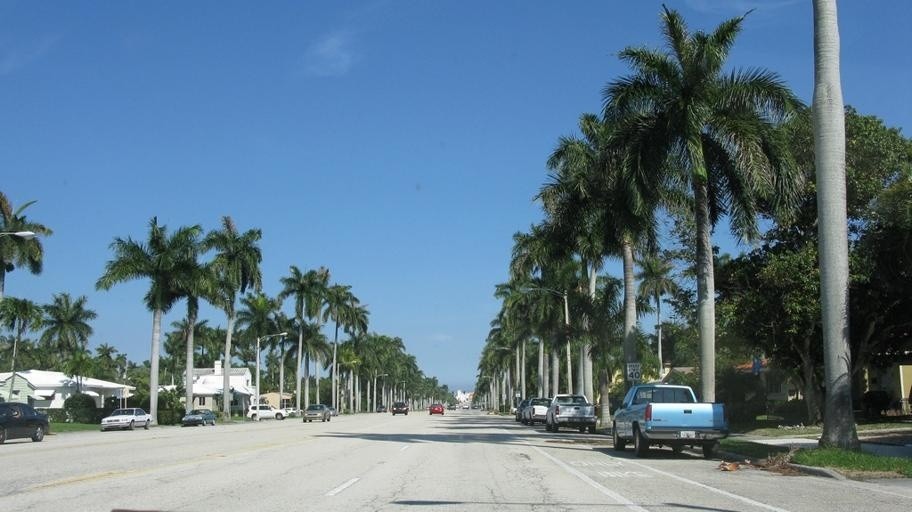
left=514, top=394, right=595, bottom=433
left=612, top=385, right=728, bottom=459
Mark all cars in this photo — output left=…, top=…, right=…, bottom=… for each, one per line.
left=0, top=402, right=48, bottom=442
left=448, top=404, right=468, bottom=410
left=303, top=404, right=338, bottom=422
left=392, top=401, right=409, bottom=415
left=377, top=406, right=387, bottom=412
left=101, top=408, right=151, bottom=430
left=430, top=404, right=444, bottom=415
left=181, top=406, right=216, bottom=426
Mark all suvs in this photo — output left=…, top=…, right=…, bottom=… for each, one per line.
left=246, top=404, right=288, bottom=421
left=282, top=408, right=303, bottom=416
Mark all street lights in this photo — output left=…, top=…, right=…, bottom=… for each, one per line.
left=257, top=332, right=288, bottom=421
left=483, top=287, right=572, bottom=412
left=373, top=369, right=406, bottom=410
left=337, top=361, right=356, bottom=412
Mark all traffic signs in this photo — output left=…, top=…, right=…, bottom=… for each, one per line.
left=627, top=363, right=641, bottom=381
